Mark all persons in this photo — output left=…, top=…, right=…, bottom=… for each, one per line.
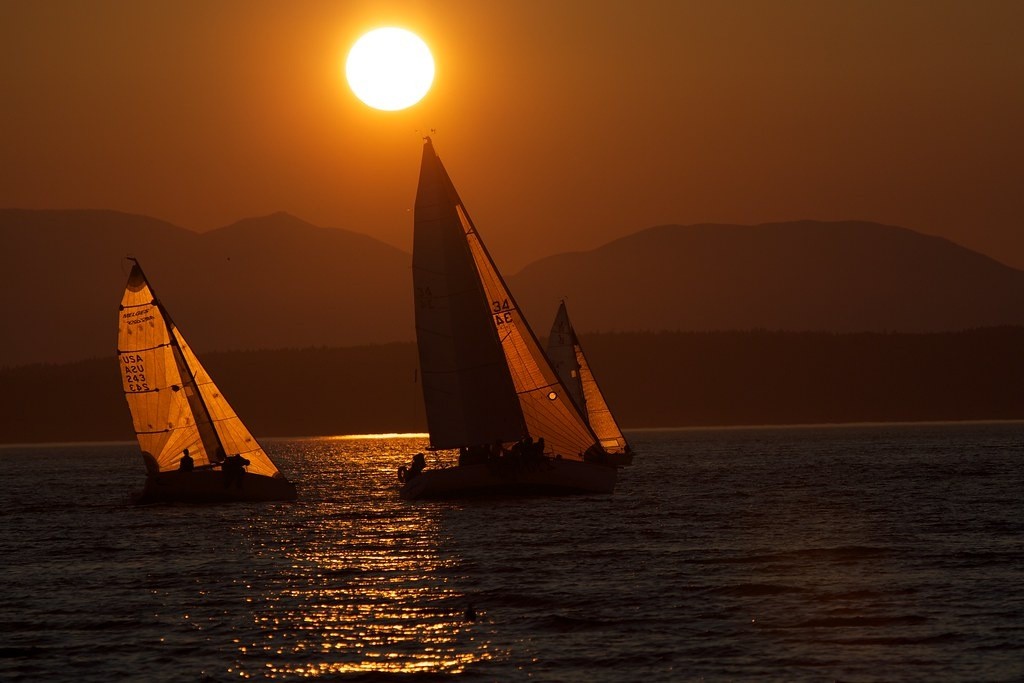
left=180, top=448, right=194, bottom=471
left=458, top=437, right=546, bottom=466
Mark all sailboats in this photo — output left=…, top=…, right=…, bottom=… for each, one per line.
left=399, top=136, right=619, bottom=501
left=503, top=297, right=633, bottom=464
left=117, top=257, right=298, bottom=505
